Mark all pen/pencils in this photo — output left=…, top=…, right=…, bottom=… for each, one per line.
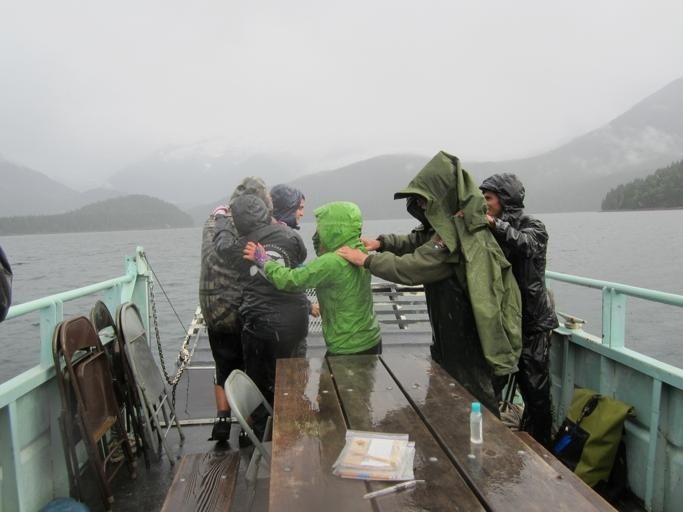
left=362, top=480, right=417, bottom=500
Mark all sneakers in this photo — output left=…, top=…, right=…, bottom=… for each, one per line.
left=212, top=424, right=252, bottom=447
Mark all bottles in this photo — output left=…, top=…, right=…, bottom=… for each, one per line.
left=470, top=402, right=482, bottom=444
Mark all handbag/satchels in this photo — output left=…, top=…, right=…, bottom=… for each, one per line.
left=552, top=389, right=637, bottom=501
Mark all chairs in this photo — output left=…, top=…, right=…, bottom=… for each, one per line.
left=224, top=369, right=273, bottom=511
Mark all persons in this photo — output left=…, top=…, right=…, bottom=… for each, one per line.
left=338, top=149, right=524, bottom=419
left=270, top=183, right=322, bottom=316
left=243, top=202, right=383, bottom=355
left=0, top=246, right=13, bottom=321
left=199, top=177, right=273, bottom=447
left=480, top=171, right=559, bottom=448
left=214, top=194, right=311, bottom=460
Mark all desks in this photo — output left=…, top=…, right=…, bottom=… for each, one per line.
left=269, top=352, right=621, bottom=512
